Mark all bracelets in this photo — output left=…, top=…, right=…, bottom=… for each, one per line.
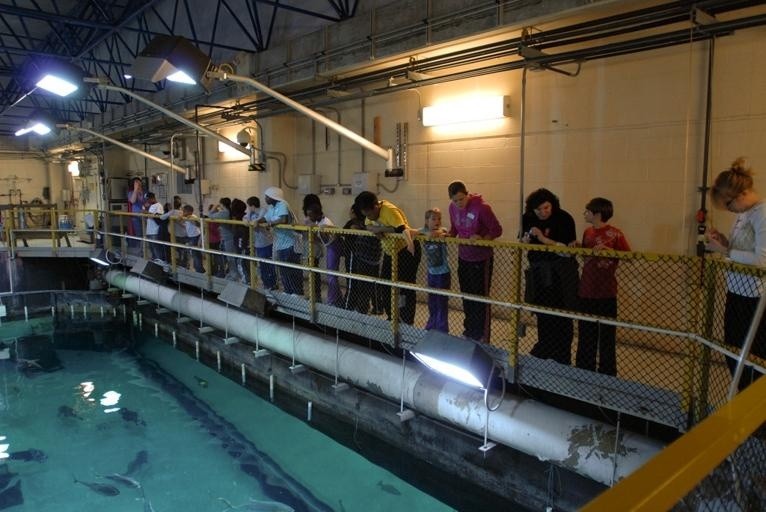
left=725, top=247, right=732, bottom=258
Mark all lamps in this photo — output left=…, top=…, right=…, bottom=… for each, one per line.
left=126, top=257, right=170, bottom=288
left=412, top=93, right=515, bottom=131
left=212, top=282, right=270, bottom=319
left=20, top=117, right=188, bottom=187
left=83, top=244, right=114, bottom=272
left=128, top=33, right=396, bottom=176
left=405, top=328, right=499, bottom=400
left=31, top=61, right=257, bottom=175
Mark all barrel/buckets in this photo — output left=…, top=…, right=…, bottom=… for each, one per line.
left=57, top=215, right=75, bottom=230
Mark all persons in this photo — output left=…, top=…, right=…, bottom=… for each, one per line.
left=129, top=176, right=503, bottom=344
left=702, top=156, right=766, bottom=445
left=517, top=188, right=631, bottom=376
